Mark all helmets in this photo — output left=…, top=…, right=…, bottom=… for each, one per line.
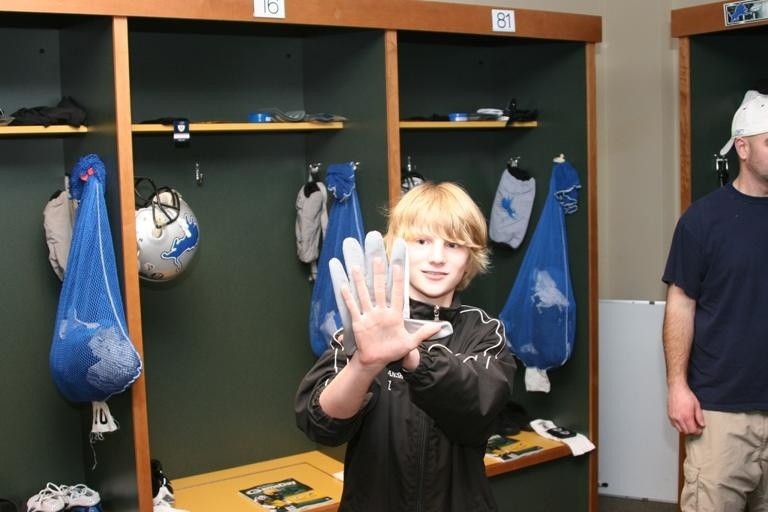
left=135, top=176, right=200, bottom=283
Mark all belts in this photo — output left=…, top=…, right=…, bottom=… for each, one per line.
left=714, top=154, right=729, bottom=189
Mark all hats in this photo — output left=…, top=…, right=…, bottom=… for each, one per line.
left=719, top=95, right=768, bottom=156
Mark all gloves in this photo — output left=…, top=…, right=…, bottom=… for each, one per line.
left=329, top=231, right=453, bottom=362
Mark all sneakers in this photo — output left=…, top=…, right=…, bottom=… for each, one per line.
left=26, top=483, right=101, bottom=511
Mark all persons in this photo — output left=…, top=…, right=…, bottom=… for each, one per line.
left=660, top=87, right=767, bottom=510
left=255, top=488, right=297, bottom=512
left=293, top=179, right=527, bottom=512
